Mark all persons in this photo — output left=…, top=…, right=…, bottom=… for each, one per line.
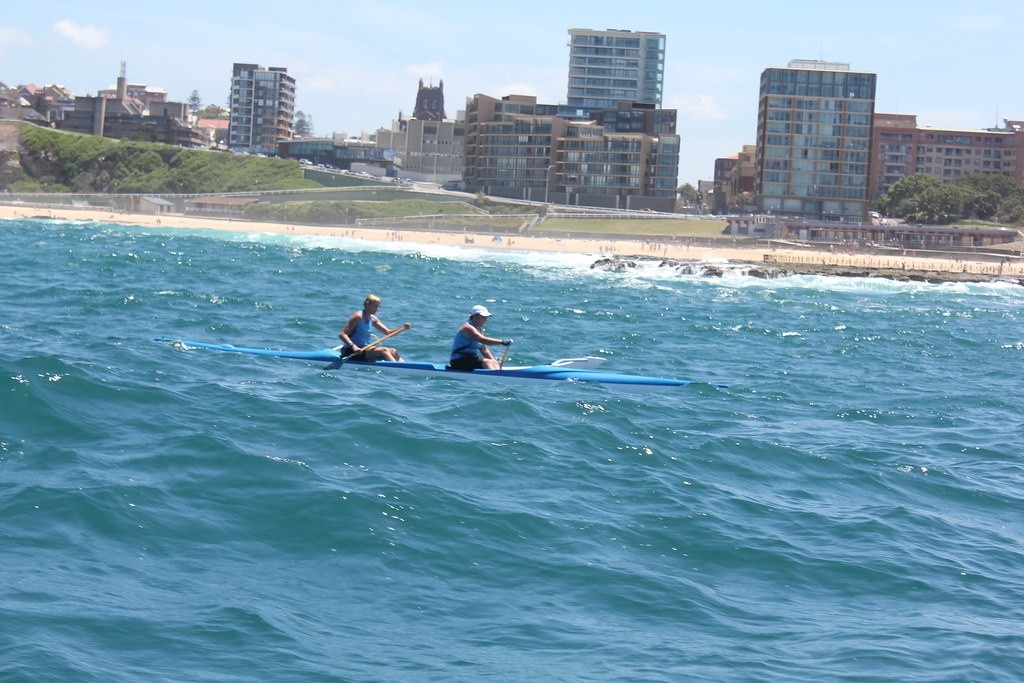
left=339, top=294, right=412, bottom=362
left=450, top=304, right=514, bottom=370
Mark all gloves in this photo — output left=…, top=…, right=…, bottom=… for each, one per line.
left=502, top=338, right=513, bottom=347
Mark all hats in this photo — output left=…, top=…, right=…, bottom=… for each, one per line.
left=469, top=305, right=492, bottom=317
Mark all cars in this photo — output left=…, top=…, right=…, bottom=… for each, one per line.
left=300, top=159, right=313, bottom=165
left=319, top=164, right=381, bottom=180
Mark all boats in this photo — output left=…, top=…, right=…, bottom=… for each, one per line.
left=155, top=337, right=730, bottom=391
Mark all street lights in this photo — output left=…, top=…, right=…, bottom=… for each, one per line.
left=345, top=208, right=348, bottom=224
left=545, top=165, right=554, bottom=202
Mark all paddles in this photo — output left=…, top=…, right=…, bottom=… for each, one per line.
left=500, top=338, right=514, bottom=367
left=323, top=327, right=406, bottom=370
